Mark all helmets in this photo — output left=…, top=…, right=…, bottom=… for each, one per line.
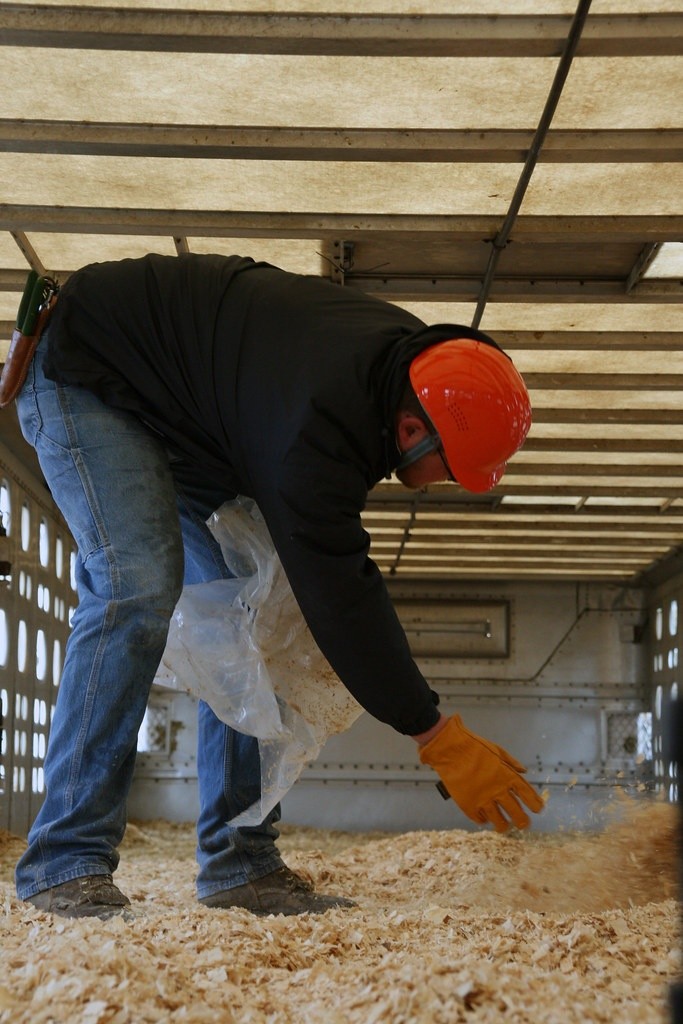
left=408, top=336, right=533, bottom=495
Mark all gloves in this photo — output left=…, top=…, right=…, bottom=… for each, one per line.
left=415, top=715, right=543, bottom=833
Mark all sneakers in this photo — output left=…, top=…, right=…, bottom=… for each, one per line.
left=198, top=857, right=358, bottom=917
left=28, top=874, right=130, bottom=923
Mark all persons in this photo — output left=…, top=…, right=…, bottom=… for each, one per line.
left=14, top=253, right=543, bottom=917
